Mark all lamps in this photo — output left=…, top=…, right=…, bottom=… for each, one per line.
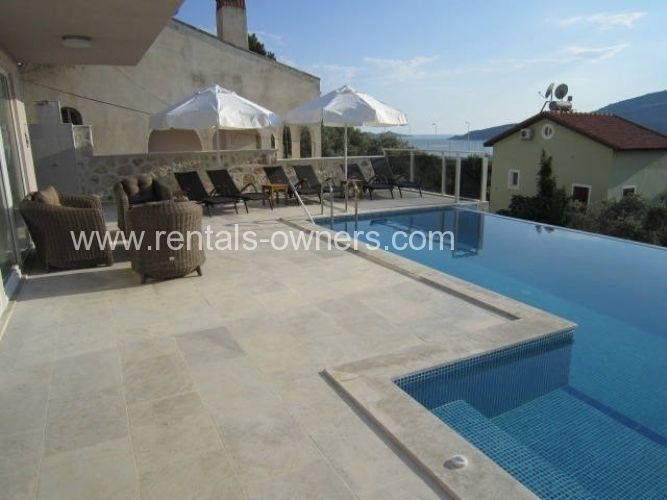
left=60, top=34, right=92, bottom=50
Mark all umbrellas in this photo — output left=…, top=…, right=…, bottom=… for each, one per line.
left=281, top=82, right=409, bottom=200
left=147, top=81, right=282, bottom=168
